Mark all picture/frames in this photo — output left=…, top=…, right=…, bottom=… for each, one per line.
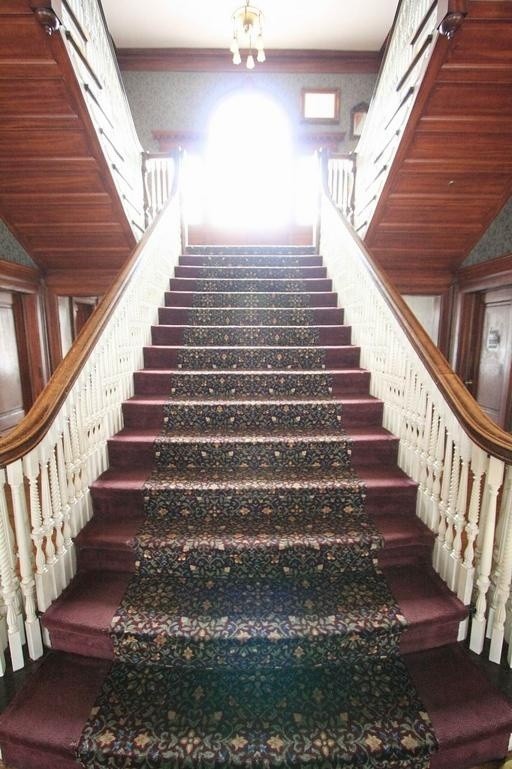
left=299, top=86, right=370, bottom=140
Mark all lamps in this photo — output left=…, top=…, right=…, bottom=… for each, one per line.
left=226, top=1, right=269, bottom=69
